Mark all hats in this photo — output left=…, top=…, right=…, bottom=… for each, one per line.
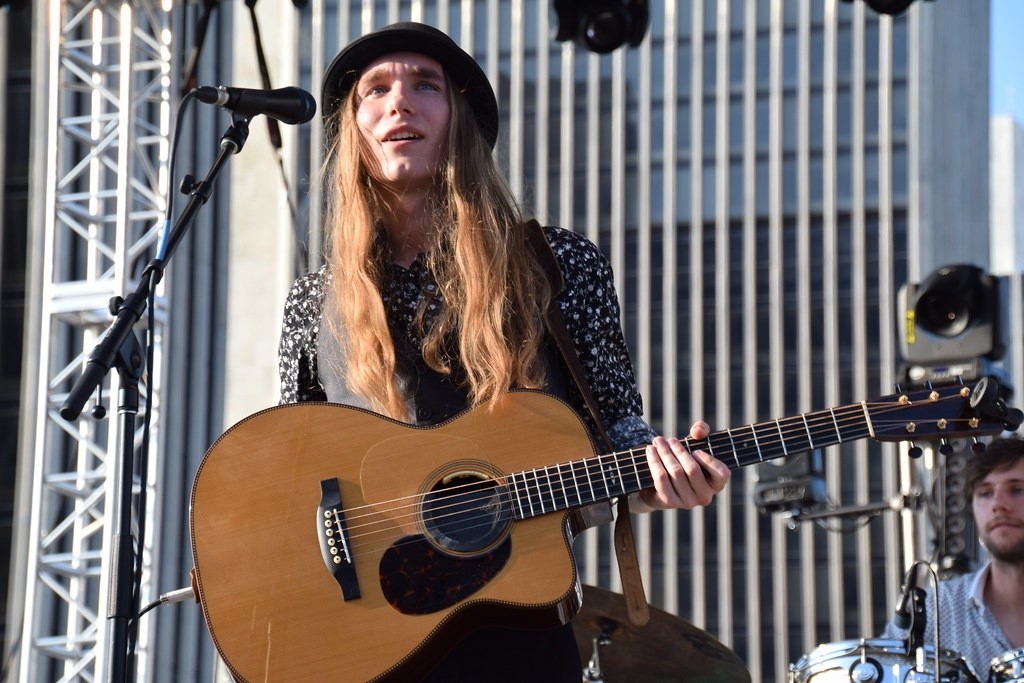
left=320, top=21, right=500, bottom=155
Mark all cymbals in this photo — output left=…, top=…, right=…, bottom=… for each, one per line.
left=566, top=580, right=752, bottom=683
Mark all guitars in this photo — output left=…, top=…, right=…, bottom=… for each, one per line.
left=189, top=368, right=1020, bottom=682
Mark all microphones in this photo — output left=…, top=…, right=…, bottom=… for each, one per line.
left=894, top=562, right=920, bottom=629
left=191, top=85, right=316, bottom=125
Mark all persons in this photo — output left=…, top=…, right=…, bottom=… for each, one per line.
left=881, top=434, right=1023, bottom=682
left=280, top=19, right=731, bottom=682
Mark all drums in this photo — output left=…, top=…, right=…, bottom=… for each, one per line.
left=785, top=637, right=981, bottom=683
